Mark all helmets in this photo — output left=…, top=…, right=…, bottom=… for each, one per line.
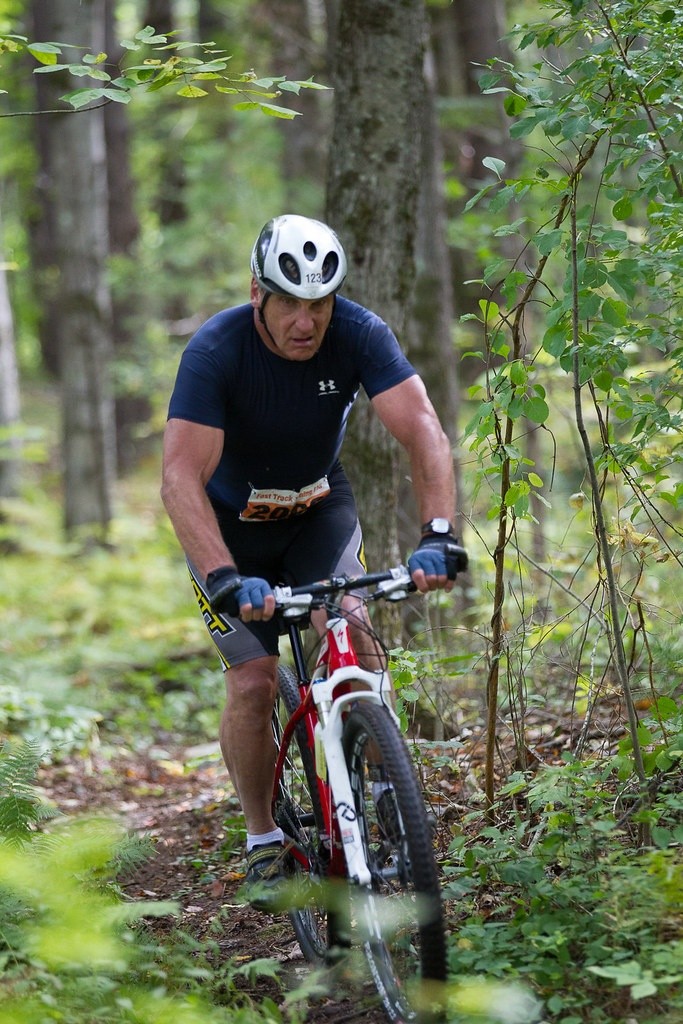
left=251, top=214, right=347, bottom=299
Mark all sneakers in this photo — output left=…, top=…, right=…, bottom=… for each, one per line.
left=245, top=840, right=294, bottom=912
left=376, top=789, right=437, bottom=849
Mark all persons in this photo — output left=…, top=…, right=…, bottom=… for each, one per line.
left=161, top=215, right=458, bottom=913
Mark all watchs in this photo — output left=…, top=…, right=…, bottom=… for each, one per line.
left=420, top=518, right=456, bottom=538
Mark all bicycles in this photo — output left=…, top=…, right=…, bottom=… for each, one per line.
left=227, top=560, right=467, bottom=1024
